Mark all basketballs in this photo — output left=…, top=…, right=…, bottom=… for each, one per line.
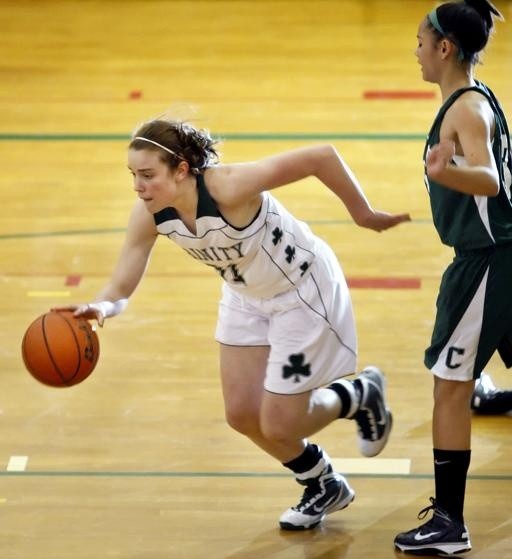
left=22, top=311, right=99, bottom=387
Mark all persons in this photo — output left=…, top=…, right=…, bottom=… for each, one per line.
left=51, top=117, right=410, bottom=532
left=391, top=0, right=512, bottom=558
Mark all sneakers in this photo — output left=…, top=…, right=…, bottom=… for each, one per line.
left=276, top=463, right=357, bottom=535
left=392, top=496, right=472, bottom=558
left=352, top=365, right=394, bottom=462
left=470, top=373, right=512, bottom=417
